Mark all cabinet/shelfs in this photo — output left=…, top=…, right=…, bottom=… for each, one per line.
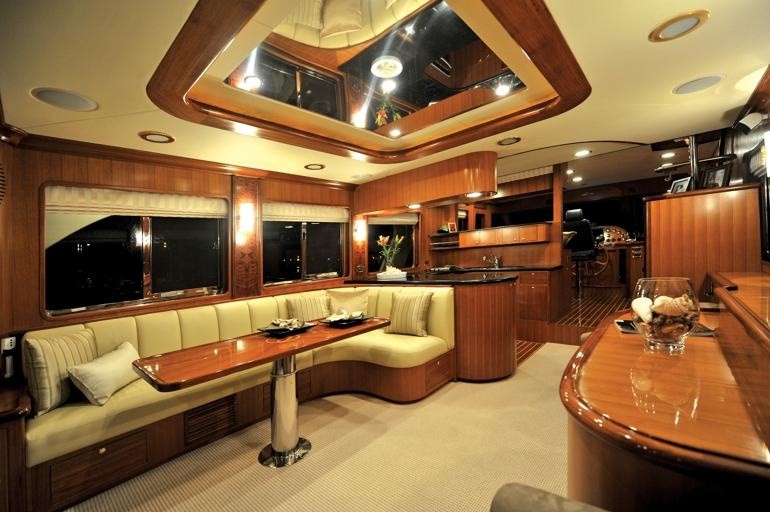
left=519, top=270, right=569, bottom=325
left=502, top=224, right=551, bottom=246
left=448, top=223, right=458, bottom=233
left=458, top=225, right=501, bottom=247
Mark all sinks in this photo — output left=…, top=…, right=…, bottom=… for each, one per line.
left=465, top=267, right=516, bottom=269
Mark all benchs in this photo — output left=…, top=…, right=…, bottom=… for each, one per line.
left=21, top=281, right=457, bottom=510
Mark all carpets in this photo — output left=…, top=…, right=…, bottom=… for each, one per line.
left=515, top=288, right=632, bottom=369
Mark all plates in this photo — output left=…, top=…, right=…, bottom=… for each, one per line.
left=257, top=319, right=317, bottom=335
left=319, top=312, right=376, bottom=328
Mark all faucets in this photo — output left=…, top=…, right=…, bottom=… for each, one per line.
left=483, top=256, right=499, bottom=268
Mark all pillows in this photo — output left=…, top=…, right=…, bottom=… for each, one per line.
left=27, top=327, right=145, bottom=417
left=286, top=288, right=435, bottom=339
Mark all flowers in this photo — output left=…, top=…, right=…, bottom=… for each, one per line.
left=375, top=91, right=402, bottom=129
left=375, top=233, right=405, bottom=268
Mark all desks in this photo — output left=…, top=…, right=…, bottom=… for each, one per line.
left=131, top=313, right=392, bottom=470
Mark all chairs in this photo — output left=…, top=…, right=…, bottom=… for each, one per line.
left=563, top=207, right=599, bottom=301
